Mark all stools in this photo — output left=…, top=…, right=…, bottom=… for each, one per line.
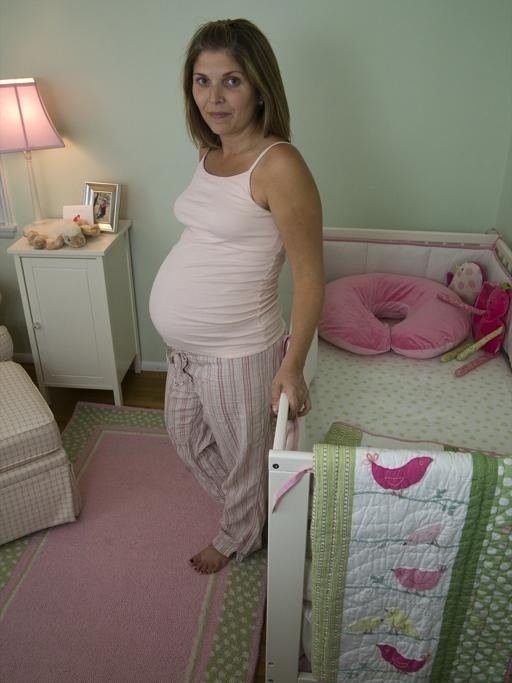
left=0, top=324, right=83, bottom=549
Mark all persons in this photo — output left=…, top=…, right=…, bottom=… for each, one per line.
left=145, top=13, right=330, bottom=577
left=96, top=196, right=108, bottom=220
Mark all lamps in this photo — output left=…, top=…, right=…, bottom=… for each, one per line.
left=0, top=75, right=65, bottom=238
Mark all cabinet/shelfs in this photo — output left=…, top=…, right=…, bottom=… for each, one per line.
left=5, top=217, right=142, bottom=408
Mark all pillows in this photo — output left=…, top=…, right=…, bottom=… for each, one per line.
left=318, top=269, right=471, bottom=359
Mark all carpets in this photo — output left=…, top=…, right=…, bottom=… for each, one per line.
left=0, top=400, right=266, bottom=683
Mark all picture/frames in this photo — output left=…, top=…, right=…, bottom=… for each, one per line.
left=80, top=179, right=121, bottom=235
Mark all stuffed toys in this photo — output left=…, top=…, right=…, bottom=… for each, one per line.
left=439, top=281, right=512, bottom=377
left=24, top=213, right=101, bottom=249
left=447, top=260, right=490, bottom=309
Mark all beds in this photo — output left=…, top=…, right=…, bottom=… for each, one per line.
left=263, top=226, right=511, bottom=683
